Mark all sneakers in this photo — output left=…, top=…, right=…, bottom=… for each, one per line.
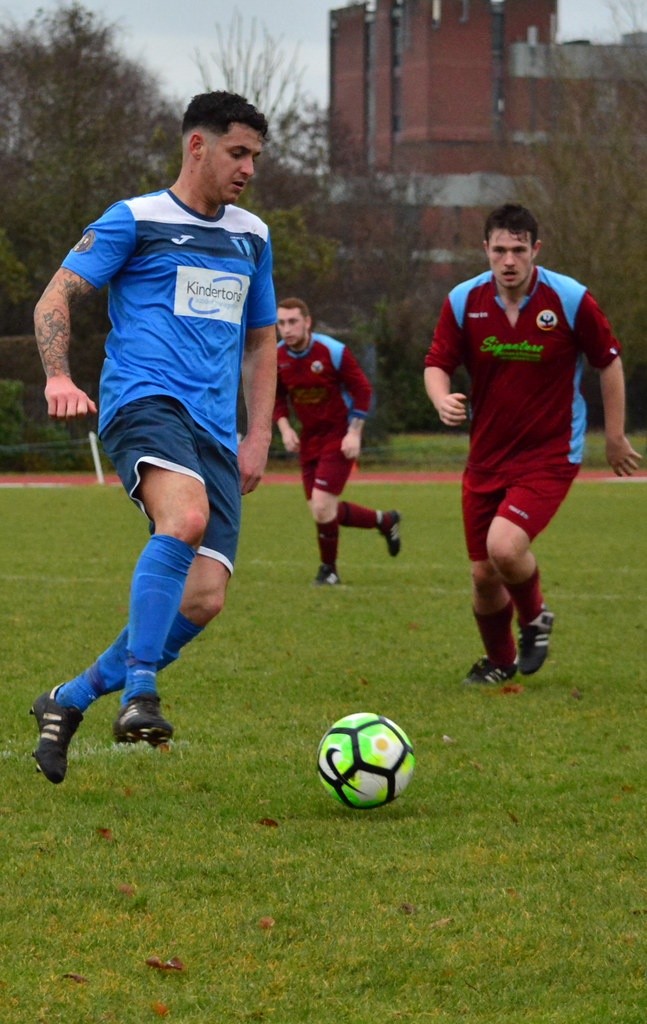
left=461, top=657, right=518, bottom=688
left=382, top=507, right=404, bottom=556
left=517, top=609, right=556, bottom=676
left=30, top=682, right=85, bottom=785
left=312, top=563, right=341, bottom=586
left=113, top=692, right=176, bottom=750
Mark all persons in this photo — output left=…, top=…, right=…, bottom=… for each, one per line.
left=30, top=92, right=280, bottom=784
left=424, top=204, right=643, bottom=684
left=275, top=298, right=401, bottom=585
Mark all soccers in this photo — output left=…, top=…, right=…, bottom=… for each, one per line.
left=315, top=712, right=416, bottom=810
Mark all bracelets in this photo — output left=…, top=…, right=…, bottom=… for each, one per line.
left=352, top=413, right=365, bottom=419
